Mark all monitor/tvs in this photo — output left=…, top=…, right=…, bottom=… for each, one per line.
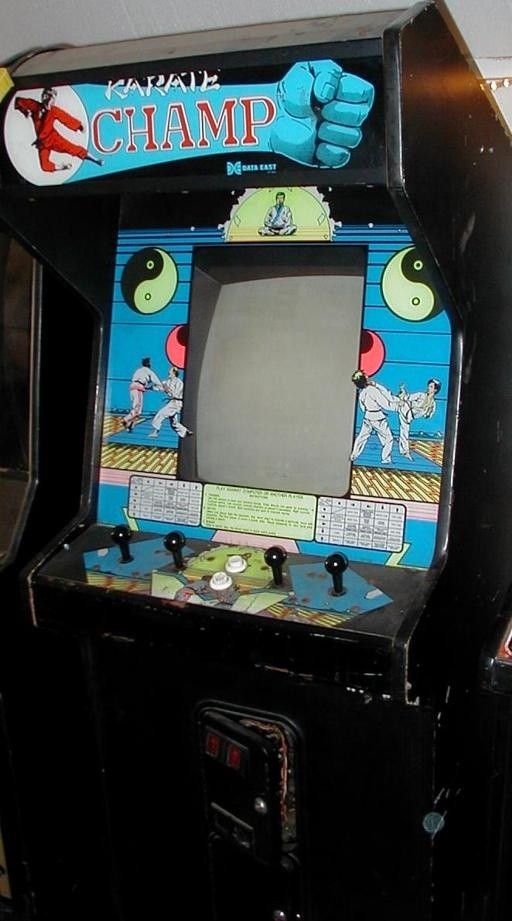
left=92, top=221, right=450, bottom=569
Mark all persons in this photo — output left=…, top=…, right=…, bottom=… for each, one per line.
left=367, top=378, right=442, bottom=462
left=120, top=357, right=164, bottom=433
left=15, top=88, right=104, bottom=172
left=349, top=369, right=404, bottom=464
left=258, top=192, right=297, bottom=236
left=146, top=366, right=194, bottom=439
